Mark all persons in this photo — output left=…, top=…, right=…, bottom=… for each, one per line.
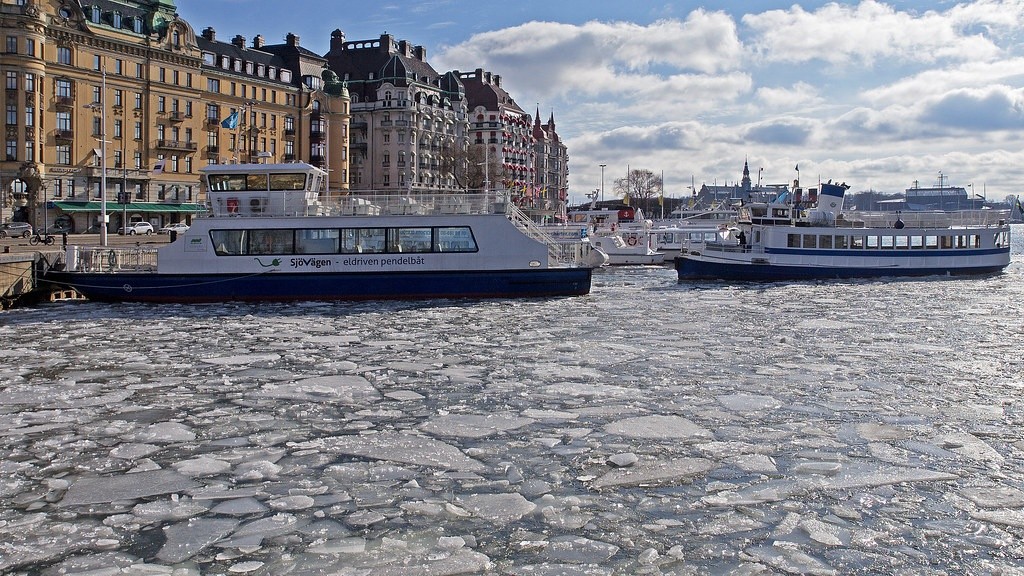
left=735, top=231, right=748, bottom=253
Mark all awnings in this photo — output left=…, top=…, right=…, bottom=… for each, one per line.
left=94, top=148, right=102, bottom=157
left=50, top=200, right=209, bottom=211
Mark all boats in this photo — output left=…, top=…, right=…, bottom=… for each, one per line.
left=906, top=171, right=986, bottom=211
left=674, top=202, right=1012, bottom=280
left=592, top=221, right=665, bottom=265
left=35, top=151, right=609, bottom=302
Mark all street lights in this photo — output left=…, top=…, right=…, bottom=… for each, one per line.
left=41, top=178, right=52, bottom=245
left=598, top=165, right=606, bottom=201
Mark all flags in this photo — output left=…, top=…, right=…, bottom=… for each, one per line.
left=1016, top=199, right=1024, bottom=214
left=221, top=111, right=238, bottom=128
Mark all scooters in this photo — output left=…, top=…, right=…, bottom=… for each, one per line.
left=30, top=229, right=55, bottom=246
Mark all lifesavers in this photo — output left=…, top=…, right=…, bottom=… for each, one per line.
left=628, top=236, right=637, bottom=246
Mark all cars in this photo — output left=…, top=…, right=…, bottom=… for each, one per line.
left=0, top=222, right=32, bottom=239
left=118, top=222, right=154, bottom=236
left=157, top=223, right=190, bottom=235
